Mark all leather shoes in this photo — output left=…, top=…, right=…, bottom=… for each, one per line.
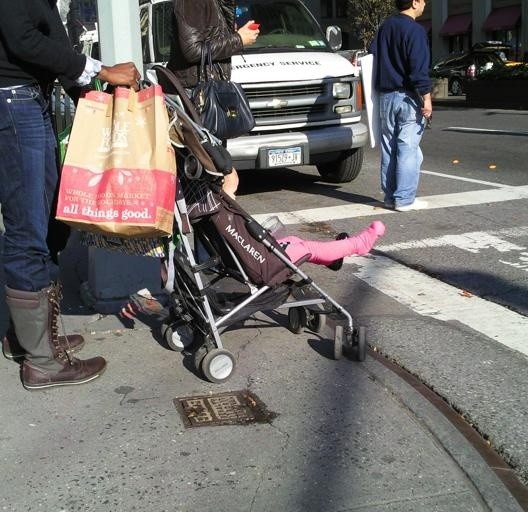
left=323, top=230, right=351, bottom=272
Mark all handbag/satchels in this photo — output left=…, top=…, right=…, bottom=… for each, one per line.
left=47, top=73, right=182, bottom=263
left=190, top=35, right=260, bottom=143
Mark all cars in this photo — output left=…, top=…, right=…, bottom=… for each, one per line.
left=432, top=42, right=527, bottom=96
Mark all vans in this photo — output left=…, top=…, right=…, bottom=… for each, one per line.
left=80, top=1, right=371, bottom=184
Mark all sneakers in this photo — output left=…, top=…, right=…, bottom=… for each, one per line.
left=382, top=197, right=395, bottom=209
left=392, top=196, right=431, bottom=212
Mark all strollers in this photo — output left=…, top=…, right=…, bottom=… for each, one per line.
left=134, top=62, right=368, bottom=384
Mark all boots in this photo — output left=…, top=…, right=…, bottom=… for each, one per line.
left=1, top=306, right=88, bottom=362
left=2, top=277, right=110, bottom=392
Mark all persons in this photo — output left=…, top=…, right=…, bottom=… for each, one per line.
left=368, top=0, right=433, bottom=212
left=223, top=167, right=385, bottom=284
left=167, top=0, right=260, bottom=149
left=0, top=0, right=142, bottom=391
